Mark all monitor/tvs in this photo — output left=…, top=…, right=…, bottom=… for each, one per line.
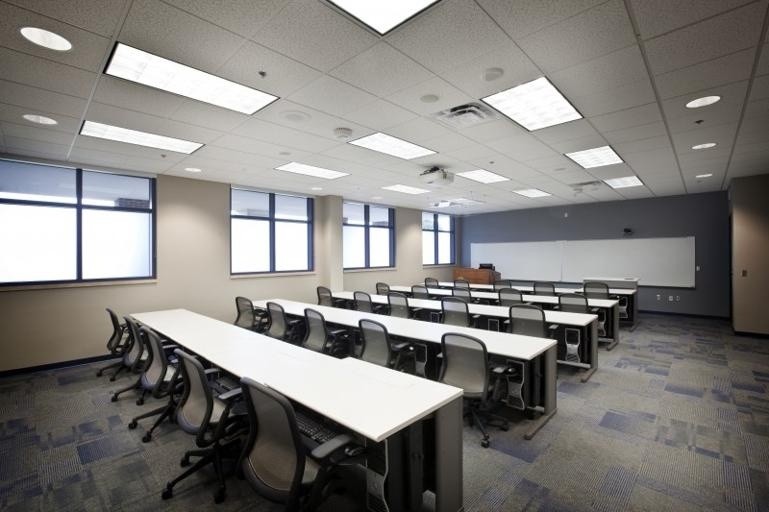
left=480, top=264, right=492, bottom=270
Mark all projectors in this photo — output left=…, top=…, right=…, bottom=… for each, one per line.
left=420, top=171, right=454, bottom=187
